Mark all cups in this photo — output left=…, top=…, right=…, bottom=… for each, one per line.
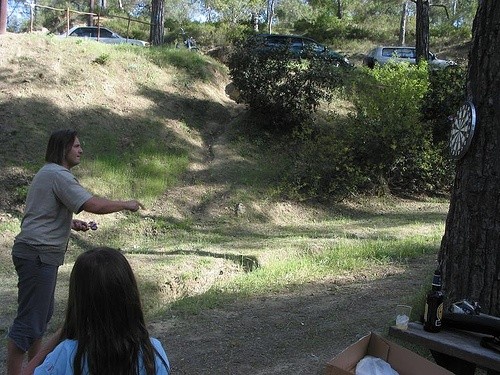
left=395, top=304, right=413, bottom=330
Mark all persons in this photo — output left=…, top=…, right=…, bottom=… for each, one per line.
left=18, top=246, right=170, bottom=375
left=7, top=128, right=145, bottom=375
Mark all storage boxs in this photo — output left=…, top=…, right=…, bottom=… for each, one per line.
left=325, top=329, right=456, bottom=375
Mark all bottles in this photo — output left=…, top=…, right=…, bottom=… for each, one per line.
left=422, top=274, right=444, bottom=333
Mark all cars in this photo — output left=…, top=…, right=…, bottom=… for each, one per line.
left=61, top=25, right=150, bottom=48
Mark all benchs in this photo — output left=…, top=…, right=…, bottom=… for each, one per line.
left=389, top=319, right=500, bottom=375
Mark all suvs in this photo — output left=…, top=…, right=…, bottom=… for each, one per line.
left=362, top=44, right=459, bottom=76
left=245, top=34, right=351, bottom=67
